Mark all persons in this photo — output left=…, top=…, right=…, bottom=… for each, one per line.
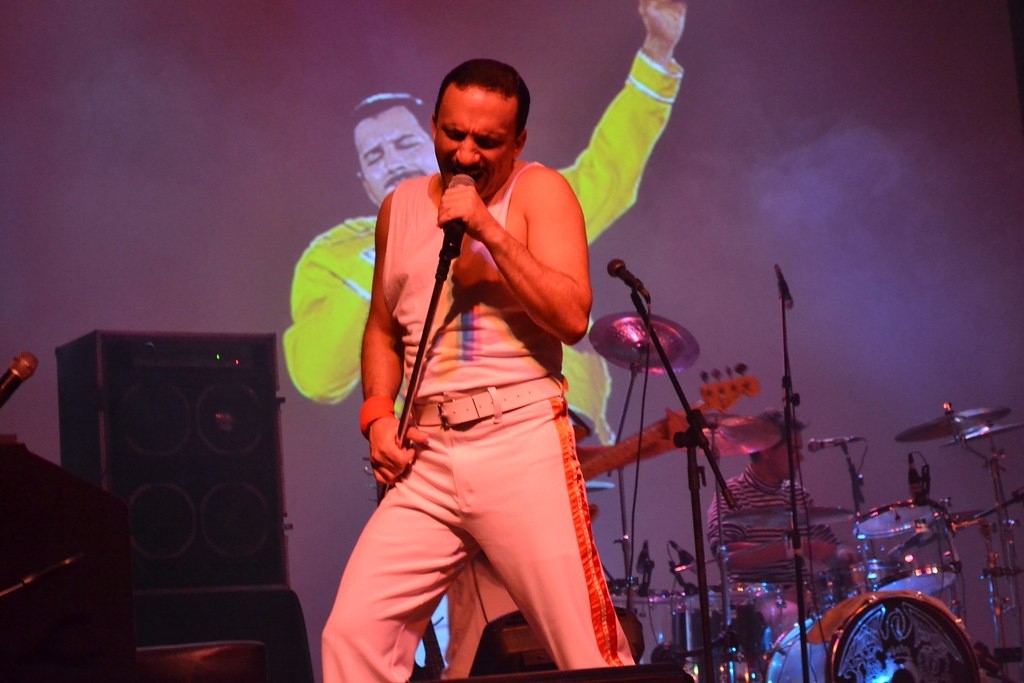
left=708, top=412, right=853, bottom=583
left=283, top=0, right=688, bottom=667
left=318, top=57, right=636, bottom=683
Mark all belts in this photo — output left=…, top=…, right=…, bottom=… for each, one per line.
left=413, top=373, right=569, bottom=425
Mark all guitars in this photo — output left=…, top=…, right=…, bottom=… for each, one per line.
left=580, top=362, right=762, bottom=481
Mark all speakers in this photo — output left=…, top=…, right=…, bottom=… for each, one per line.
left=468, top=606, right=645, bottom=672
left=54, top=329, right=287, bottom=591
left=134, top=585, right=315, bottom=683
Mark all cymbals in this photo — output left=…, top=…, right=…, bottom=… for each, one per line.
left=587, top=310, right=701, bottom=376
left=940, top=421, right=1024, bottom=448
left=719, top=502, right=856, bottom=527
left=678, top=413, right=782, bottom=458
left=893, top=405, right=1012, bottom=443
left=585, top=482, right=616, bottom=493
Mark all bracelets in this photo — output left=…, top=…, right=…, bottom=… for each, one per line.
left=783, top=539, right=805, bottom=561
left=360, top=396, right=395, bottom=440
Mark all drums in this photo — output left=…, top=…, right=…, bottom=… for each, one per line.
left=671, top=582, right=786, bottom=683
left=765, top=589, right=980, bottom=683
left=856, top=497, right=960, bottom=597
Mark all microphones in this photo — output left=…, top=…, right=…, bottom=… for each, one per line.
left=0, top=352, right=39, bottom=409
left=669, top=539, right=695, bottom=566
left=607, top=258, right=651, bottom=303
left=884, top=532, right=925, bottom=565
left=441, top=172, right=477, bottom=258
left=636, top=541, right=649, bottom=574
left=808, top=436, right=862, bottom=452
left=908, top=454, right=923, bottom=499
left=775, top=266, right=794, bottom=309
